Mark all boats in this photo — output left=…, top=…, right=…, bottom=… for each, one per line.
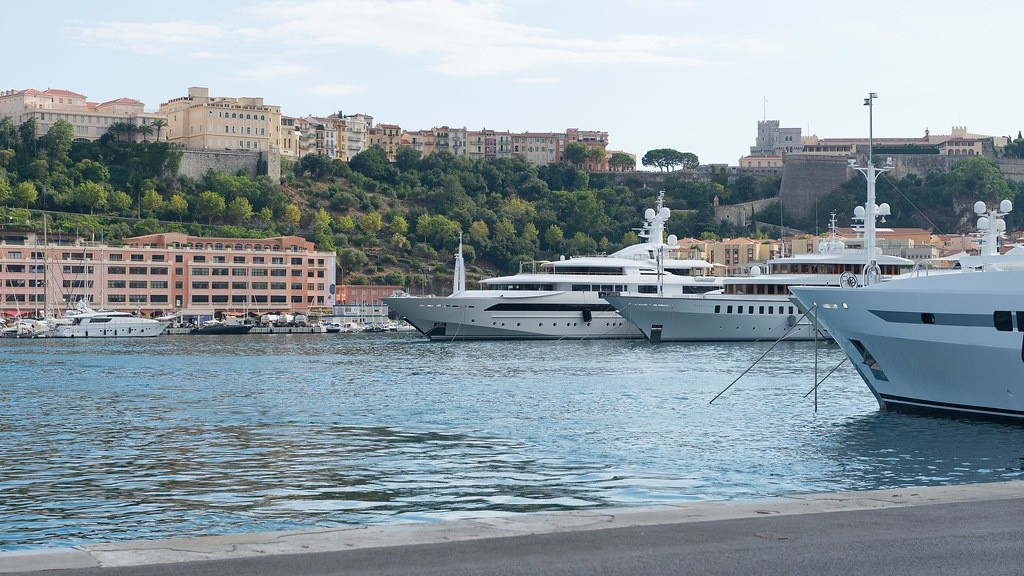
left=598, top=156, right=977, bottom=343
left=191, top=320, right=254, bottom=334
left=379, top=189, right=730, bottom=342
left=788, top=201, right=1023, bottom=424
left=48, top=299, right=173, bottom=337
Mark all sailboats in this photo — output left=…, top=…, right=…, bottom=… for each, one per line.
left=0, top=239, right=56, bottom=337
left=318, top=301, right=417, bottom=332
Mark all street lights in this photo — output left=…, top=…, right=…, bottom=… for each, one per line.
left=338, top=264, right=343, bottom=285
left=418, top=269, right=424, bottom=296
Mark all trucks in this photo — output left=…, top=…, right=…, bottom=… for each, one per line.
left=277, top=314, right=294, bottom=327
left=260, top=315, right=278, bottom=326
left=294, top=315, right=307, bottom=327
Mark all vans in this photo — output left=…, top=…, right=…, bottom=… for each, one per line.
left=203, top=321, right=218, bottom=327
